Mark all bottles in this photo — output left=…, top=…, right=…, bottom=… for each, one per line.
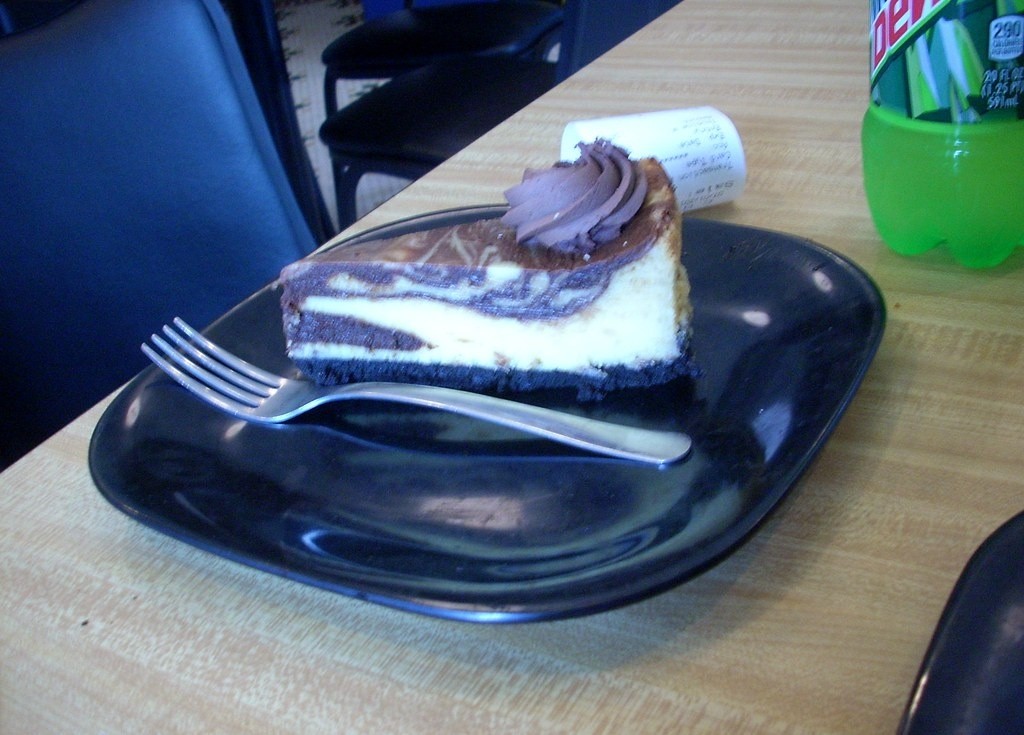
left=856, top=0, right=1024, bottom=272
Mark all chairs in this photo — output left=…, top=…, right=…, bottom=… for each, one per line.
left=0, top=0, right=337, bottom=474
left=319, top=0, right=584, bottom=232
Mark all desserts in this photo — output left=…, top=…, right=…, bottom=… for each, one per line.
left=278, top=138, right=693, bottom=397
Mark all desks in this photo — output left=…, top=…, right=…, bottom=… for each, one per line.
left=0, top=0, right=1024, bottom=735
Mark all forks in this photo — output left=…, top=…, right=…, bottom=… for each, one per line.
left=136, top=313, right=694, bottom=468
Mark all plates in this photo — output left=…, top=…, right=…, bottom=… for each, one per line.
left=895, top=505, right=1024, bottom=735
left=86, top=199, right=887, bottom=623
